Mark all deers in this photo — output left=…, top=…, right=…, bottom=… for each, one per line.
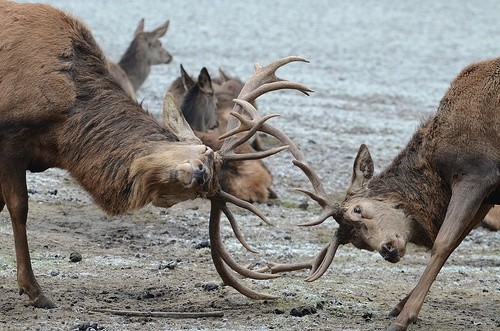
left=0, top=0, right=500, bottom=330
